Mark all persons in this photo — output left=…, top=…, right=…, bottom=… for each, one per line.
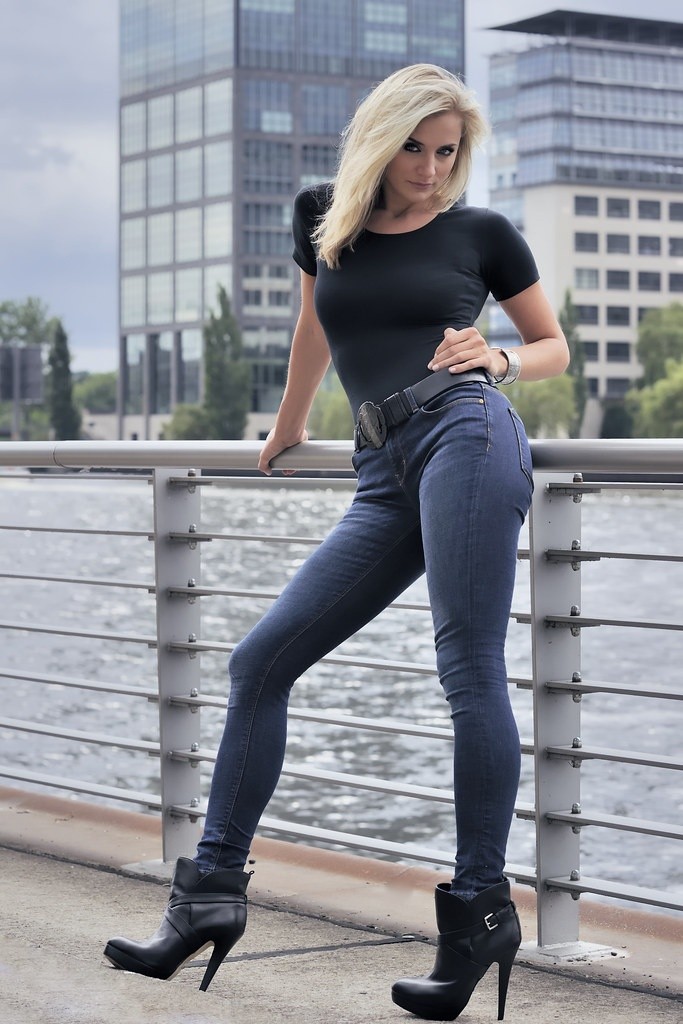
left=101, top=62, right=570, bottom=1022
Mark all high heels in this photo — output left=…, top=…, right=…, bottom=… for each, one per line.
left=103, top=856, right=254, bottom=992
left=391, top=874, right=521, bottom=1021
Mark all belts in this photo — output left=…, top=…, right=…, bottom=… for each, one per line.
left=354, top=365, right=488, bottom=451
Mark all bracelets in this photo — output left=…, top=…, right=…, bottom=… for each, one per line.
left=491, top=347, right=521, bottom=386
